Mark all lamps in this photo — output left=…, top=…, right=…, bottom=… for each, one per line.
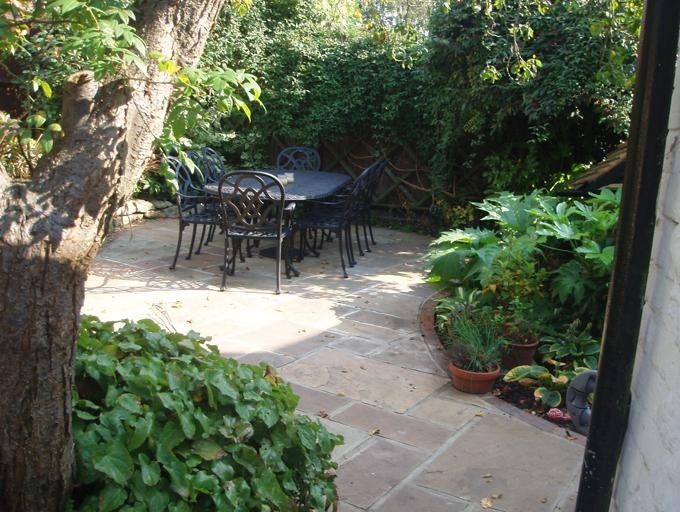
left=162, top=141, right=403, bottom=294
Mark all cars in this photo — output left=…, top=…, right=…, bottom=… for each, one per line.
left=277, top=172, right=294, bottom=186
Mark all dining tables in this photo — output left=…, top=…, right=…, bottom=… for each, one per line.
left=440, top=251, right=542, bottom=396
